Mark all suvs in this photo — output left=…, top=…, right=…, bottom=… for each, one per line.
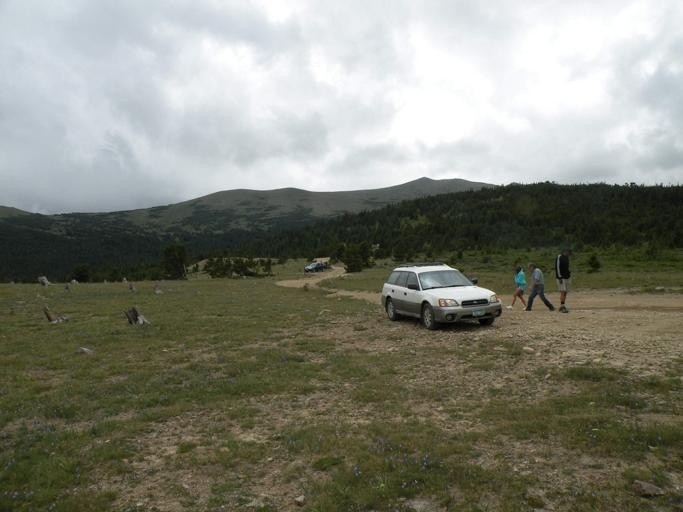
left=380, top=263, right=503, bottom=330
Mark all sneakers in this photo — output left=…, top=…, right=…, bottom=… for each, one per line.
left=506, top=305, right=569, bottom=313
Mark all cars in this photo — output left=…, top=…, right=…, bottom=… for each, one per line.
left=304, top=263, right=326, bottom=272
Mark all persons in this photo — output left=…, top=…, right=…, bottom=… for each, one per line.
left=555, top=244, right=573, bottom=313
left=505, top=264, right=527, bottom=310
left=523, top=264, right=555, bottom=312
